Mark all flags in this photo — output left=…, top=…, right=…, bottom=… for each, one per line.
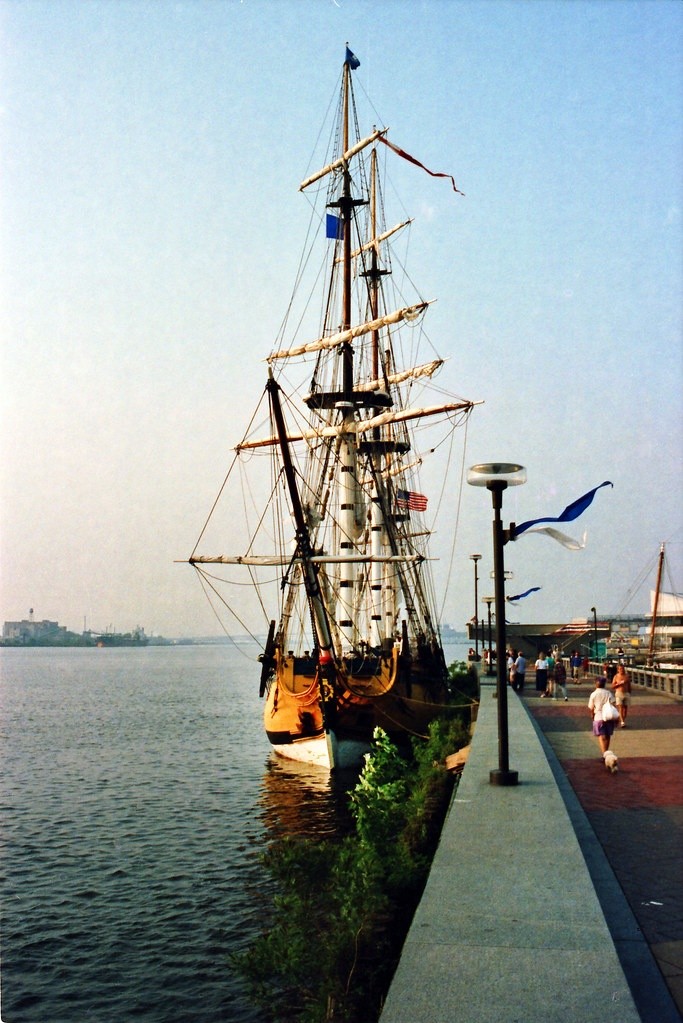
left=346, top=47, right=360, bottom=70
left=395, top=489, right=427, bottom=511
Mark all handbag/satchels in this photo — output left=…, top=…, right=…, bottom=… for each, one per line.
left=601, top=691, right=619, bottom=721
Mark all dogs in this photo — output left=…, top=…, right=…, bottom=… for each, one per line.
left=602, top=751, right=619, bottom=775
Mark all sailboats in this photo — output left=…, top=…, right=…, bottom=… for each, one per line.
left=171, top=37, right=483, bottom=781
left=559, top=539, right=683, bottom=688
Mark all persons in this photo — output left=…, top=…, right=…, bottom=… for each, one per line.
left=588, top=677, right=616, bottom=762
left=612, top=664, right=632, bottom=728
left=618, top=648, right=624, bottom=656
left=469, top=647, right=497, bottom=660
left=535, top=652, right=568, bottom=701
left=603, top=663, right=608, bottom=675
left=506, top=648, right=526, bottom=692
left=570, top=650, right=591, bottom=684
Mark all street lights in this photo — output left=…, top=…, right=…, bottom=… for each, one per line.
left=465, top=462, right=530, bottom=787
left=470, top=554, right=483, bottom=661
left=481, top=595, right=497, bottom=676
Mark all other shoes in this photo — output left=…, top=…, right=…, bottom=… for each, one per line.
left=620, top=721, right=626, bottom=728
left=549, top=693, right=554, bottom=698
left=600, top=756, right=606, bottom=761
left=552, top=698, right=557, bottom=701
left=541, top=693, right=545, bottom=697
left=565, top=697, right=568, bottom=701
left=546, top=690, right=549, bottom=695
